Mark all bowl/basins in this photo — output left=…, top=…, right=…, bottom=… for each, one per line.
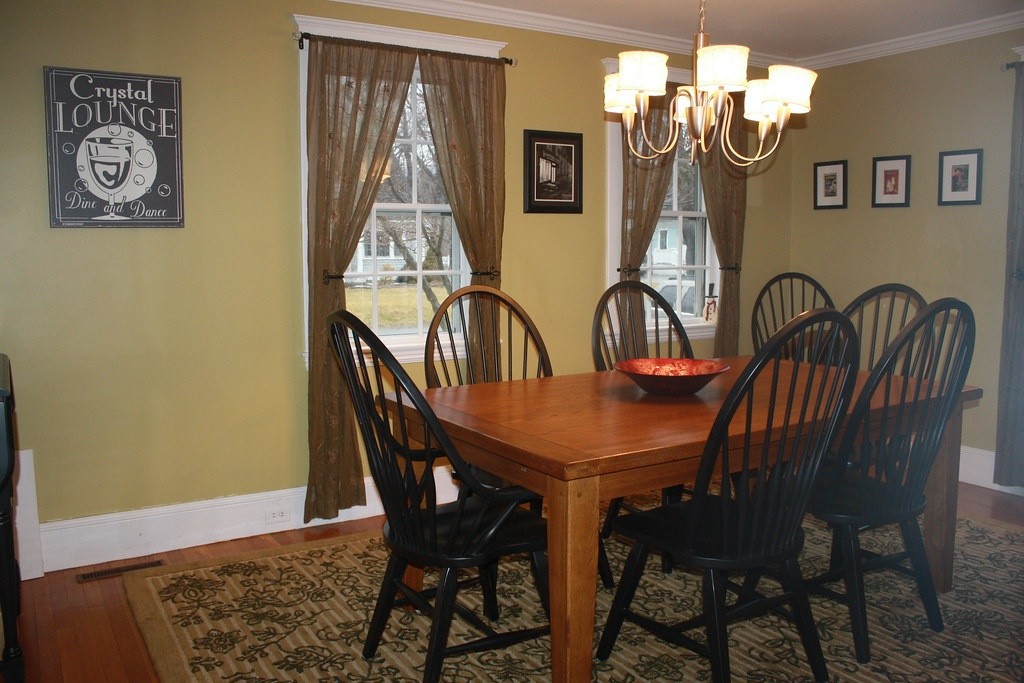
left=612, top=357, right=732, bottom=393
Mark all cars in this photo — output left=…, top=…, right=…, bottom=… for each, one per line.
left=645, top=275, right=695, bottom=320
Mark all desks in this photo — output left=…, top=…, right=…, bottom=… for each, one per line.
left=372, top=353, right=985, bottom=683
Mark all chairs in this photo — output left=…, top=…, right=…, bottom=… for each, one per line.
left=750, top=270, right=838, bottom=471
left=597, top=309, right=865, bottom=682
left=736, top=296, right=983, bottom=662
left=324, top=305, right=552, bottom=683
left=424, top=283, right=610, bottom=587
left=825, top=282, right=934, bottom=479
left=588, top=279, right=697, bottom=537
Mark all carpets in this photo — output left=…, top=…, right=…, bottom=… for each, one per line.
left=119, top=460, right=1023, bottom=683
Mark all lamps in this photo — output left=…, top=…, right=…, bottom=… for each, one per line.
left=604, top=0, right=818, bottom=167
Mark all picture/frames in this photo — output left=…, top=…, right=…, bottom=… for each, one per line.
left=938, top=148, right=983, bottom=206
left=813, top=159, right=847, bottom=209
left=872, top=154, right=909, bottom=207
left=522, top=128, right=583, bottom=213
